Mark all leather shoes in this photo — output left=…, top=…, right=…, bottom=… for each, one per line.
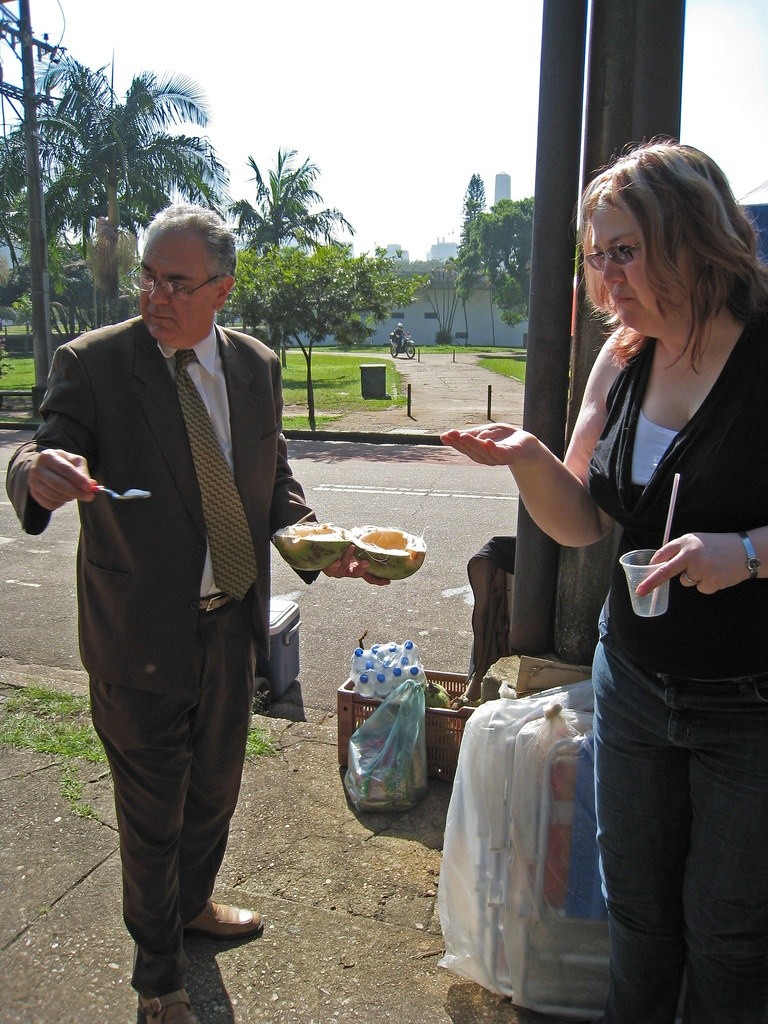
left=184, top=900, right=263, bottom=936
left=139, top=988, right=199, bottom=1024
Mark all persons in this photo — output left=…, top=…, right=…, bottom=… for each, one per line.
left=392, top=322, right=406, bottom=350
left=440, top=137, right=767, bottom=1022
left=4, top=204, right=390, bottom=1024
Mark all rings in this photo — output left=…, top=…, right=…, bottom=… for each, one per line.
left=683, top=572, right=695, bottom=584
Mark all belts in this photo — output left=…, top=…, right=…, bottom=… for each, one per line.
left=198, top=590, right=233, bottom=611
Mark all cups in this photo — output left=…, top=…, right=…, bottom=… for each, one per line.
left=619, top=549, right=669, bottom=617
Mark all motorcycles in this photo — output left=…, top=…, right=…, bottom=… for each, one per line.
left=389, top=330, right=415, bottom=359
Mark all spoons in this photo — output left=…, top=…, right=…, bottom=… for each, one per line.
left=100, top=488, right=150, bottom=499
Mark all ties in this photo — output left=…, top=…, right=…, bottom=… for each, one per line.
left=174, top=347, right=258, bottom=602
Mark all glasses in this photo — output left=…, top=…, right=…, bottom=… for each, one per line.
left=127, top=265, right=221, bottom=301
left=584, top=240, right=645, bottom=272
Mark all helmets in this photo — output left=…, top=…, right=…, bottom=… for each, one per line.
left=397, top=323, right=403, bottom=331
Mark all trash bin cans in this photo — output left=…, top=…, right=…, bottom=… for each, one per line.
left=359, top=364, right=387, bottom=400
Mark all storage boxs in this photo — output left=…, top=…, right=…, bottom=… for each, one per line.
left=335, top=666, right=472, bottom=786
left=254, top=597, right=302, bottom=703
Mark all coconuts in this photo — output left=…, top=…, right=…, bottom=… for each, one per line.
left=271, top=522, right=427, bottom=580
left=422, top=680, right=449, bottom=707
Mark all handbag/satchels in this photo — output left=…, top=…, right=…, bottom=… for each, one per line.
left=344, top=679, right=429, bottom=814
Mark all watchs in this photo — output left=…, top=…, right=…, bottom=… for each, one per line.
left=738, top=531, right=761, bottom=579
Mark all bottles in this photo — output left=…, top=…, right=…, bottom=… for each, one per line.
left=351, top=640, right=427, bottom=697
